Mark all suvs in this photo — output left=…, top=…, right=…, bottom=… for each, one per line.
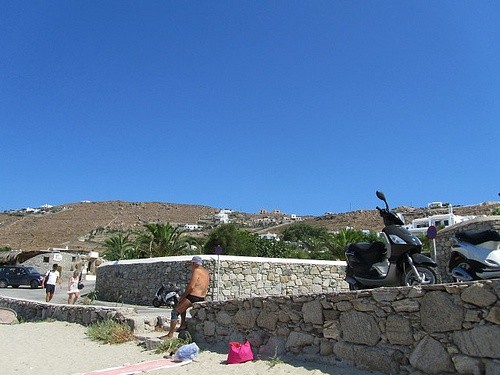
left=0, top=265, right=48, bottom=289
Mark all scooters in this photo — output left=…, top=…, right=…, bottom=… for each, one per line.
left=152, top=283, right=181, bottom=309
left=343, top=190, right=442, bottom=291
left=448, top=228, right=500, bottom=281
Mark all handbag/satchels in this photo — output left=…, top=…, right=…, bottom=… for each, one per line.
left=171, top=342, right=199, bottom=361
left=78, top=282, right=84, bottom=289
left=226, top=339, right=254, bottom=363
left=44, top=272, right=50, bottom=287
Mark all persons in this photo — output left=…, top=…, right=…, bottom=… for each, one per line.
left=67, top=270, right=83, bottom=305
left=57, top=266, right=63, bottom=289
left=159, top=256, right=209, bottom=340
left=74, top=264, right=82, bottom=289
left=42, top=263, right=61, bottom=302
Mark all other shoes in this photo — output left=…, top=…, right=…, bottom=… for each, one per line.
left=159, top=335, right=174, bottom=340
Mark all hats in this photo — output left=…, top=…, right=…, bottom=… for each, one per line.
left=52, top=263, right=59, bottom=268
left=191, top=256, right=202, bottom=266
